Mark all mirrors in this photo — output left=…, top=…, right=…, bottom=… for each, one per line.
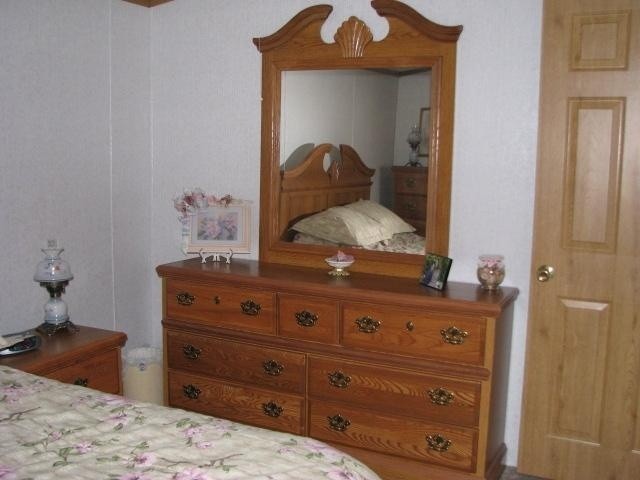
left=252, top=0, right=463, bottom=277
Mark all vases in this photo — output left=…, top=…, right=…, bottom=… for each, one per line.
left=476, top=254, right=506, bottom=291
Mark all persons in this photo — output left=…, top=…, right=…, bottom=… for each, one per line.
left=424, top=262, right=434, bottom=285
left=428, top=259, right=441, bottom=287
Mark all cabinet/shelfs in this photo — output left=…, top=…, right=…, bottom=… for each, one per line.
left=390, top=164, right=427, bottom=236
left=156, top=257, right=519, bottom=480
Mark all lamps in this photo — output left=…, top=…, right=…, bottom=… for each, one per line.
left=403, top=123, right=423, bottom=168
left=34, top=238, right=82, bottom=340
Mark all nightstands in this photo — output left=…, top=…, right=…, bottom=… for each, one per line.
left=0, top=323, right=128, bottom=397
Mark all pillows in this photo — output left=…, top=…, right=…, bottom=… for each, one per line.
left=344, top=199, right=418, bottom=235
left=288, top=206, right=393, bottom=247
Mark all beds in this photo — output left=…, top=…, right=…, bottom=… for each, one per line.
left=0, top=364, right=382, bottom=480
left=279, top=142, right=427, bottom=255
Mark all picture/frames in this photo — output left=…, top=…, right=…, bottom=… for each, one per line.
left=171, top=184, right=252, bottom=256
left=416, top=107, right=431, bottom=156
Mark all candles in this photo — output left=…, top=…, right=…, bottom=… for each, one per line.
left=327, top=249, right=353, bottom=262
